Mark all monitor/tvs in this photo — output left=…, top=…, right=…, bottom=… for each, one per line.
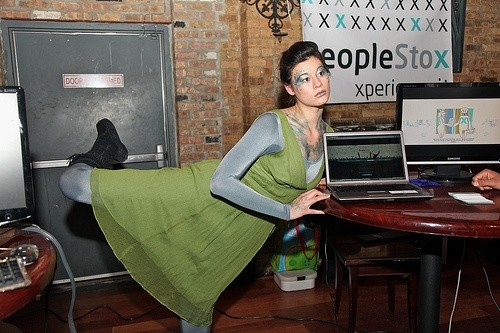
left=0, top=85, right=37, bottom=217
left=396, top=81, right=500, bottom=181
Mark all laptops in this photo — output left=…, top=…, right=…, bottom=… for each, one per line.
left=322, top=130, right=433, bottom=204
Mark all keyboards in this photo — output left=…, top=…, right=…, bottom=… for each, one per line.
left=0, top=260, right=30, bottom=292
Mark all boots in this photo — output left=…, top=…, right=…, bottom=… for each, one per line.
left=65, top=118, right=129, bottom=170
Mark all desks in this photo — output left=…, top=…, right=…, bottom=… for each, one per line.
left=0, top=234, right=57, bottom=320
left=324, top=182, right=500, bottom=333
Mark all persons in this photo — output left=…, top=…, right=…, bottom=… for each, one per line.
left=472, top=168, right=500, bottom=191
left=59, top=41, right=331, bottom=333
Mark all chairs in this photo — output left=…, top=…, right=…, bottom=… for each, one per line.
left=327, top=220, right=420, bottom=333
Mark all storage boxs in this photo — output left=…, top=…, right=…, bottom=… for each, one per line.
left=274, top=267, right=318, bottom=292
